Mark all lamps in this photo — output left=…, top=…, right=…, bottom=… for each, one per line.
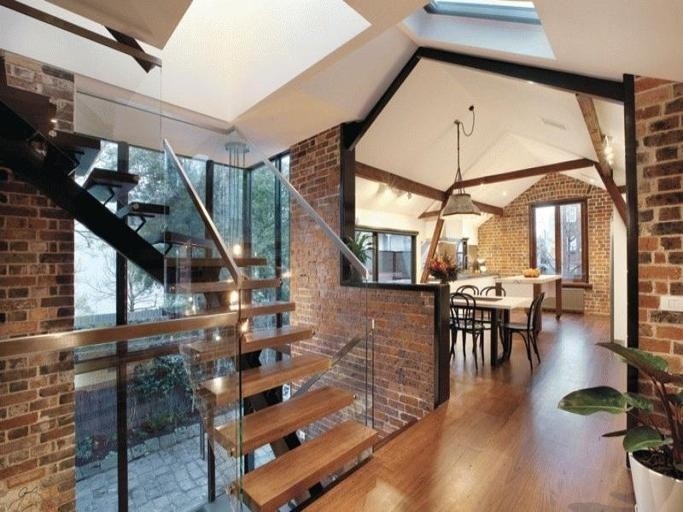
left=599, top=134, right=615, bottom=168
left=439, top=104, right=481, bottom=219
left=224, top=141, right=251, bottom=334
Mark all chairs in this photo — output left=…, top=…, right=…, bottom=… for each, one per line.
left=496, top=291, right=545, bottom=370
left=472, top=287, right=506, bottom=353
left=455, top=285, right=483, bottom=351
left=449, top=292, right=485, bottom=369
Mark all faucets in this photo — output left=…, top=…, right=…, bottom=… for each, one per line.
left=463, top=254, right=472, bottom=272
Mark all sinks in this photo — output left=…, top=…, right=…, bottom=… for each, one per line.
left=458, top=273, right=483, bottom=279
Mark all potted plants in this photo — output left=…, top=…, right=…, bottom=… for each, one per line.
left=346, top=232, right=374, bottom=281
left=557, top=339, right=682, bottom=511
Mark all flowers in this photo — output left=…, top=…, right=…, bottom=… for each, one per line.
left=424, top=251, right=459, bottom=283
